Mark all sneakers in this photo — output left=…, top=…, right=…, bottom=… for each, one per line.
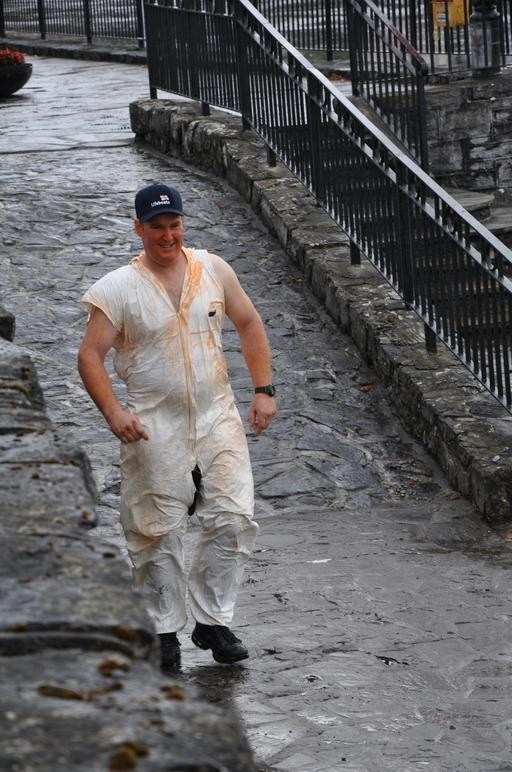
left=163, top=633, right=181, bottom=667
left=192, top=624, right=248, bottom=662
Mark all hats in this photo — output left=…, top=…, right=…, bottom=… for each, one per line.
left=135, top=185, right=184, bottom=222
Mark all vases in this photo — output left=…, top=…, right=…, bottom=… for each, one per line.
left=0, top=63, right=31, bottom=99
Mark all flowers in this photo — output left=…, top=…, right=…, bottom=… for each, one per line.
left=0, top=47, right=25, bottom=63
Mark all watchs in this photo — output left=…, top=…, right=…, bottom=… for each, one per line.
left=255, top=384, right=276, bottom=397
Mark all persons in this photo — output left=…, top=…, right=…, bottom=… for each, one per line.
left=76, top=185, right=278, bottom=678
